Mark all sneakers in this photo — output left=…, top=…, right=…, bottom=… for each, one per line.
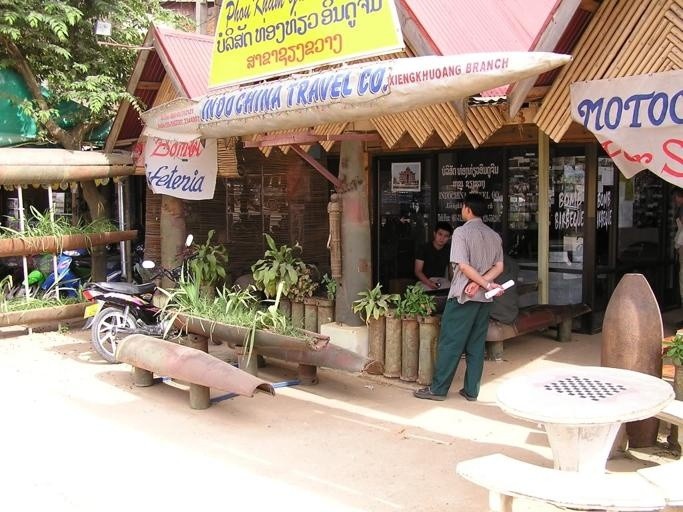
left=414, top=386, right=446, bottom=401
left=460, top=388, right=477, bottom=401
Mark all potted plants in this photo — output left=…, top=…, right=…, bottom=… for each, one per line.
left=253, top=234, right=335, bottom=336
left=183, top=228, right=229, bottom=302
left=352, top=285, right=438, bottom=385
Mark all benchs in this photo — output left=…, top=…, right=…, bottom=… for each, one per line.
left=457, top=401, right=683, bottom=512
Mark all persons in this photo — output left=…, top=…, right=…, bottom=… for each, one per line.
left=668, top=184, right=682, bottom=330
left=412, top=221, right=453, bottom=295
left=484, top=226, right=520, bottom=363
left=413, top=193, right=505, bottom=401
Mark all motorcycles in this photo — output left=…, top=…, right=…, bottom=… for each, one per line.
left=81, top=232, right=199, bottom=363
left=39, top=248, right=143, bottom=300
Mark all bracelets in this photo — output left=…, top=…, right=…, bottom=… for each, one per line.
left=485, top=282, right=491, bottom=291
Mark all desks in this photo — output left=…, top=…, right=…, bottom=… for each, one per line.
left=494, top=365, right=676, bottom=476
